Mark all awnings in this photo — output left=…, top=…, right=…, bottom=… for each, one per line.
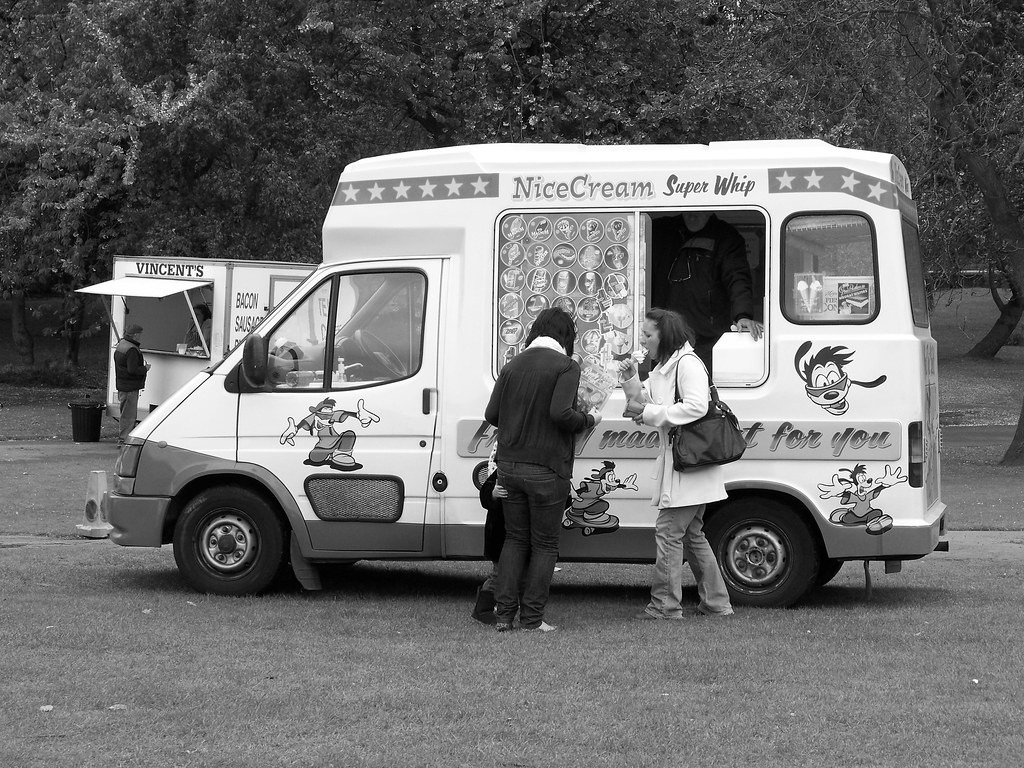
left=74, top=277, right=214, bottom=356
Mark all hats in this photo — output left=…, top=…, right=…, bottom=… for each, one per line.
left=126, top=324, right=144, bottom=334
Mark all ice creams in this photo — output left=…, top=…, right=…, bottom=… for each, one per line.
left=798, top=280, right=820, bottom=313
left=617, top=348, right=648, bottom=374
left=615, top=305, right=625, bottom=327
left=614, top=331, right=625, bottom=354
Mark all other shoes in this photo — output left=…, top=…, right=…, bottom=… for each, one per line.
left=498, top=623, right=515, bottom=631
left=694, top=607, right=735, bottom=616
left=632, top=611, right=657, bottom=620
left=522, top=620, right=558, bottom=632
left=117, top=442, right=124, bottom=446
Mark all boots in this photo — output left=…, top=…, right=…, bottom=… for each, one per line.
left=470, top=586, right=499, bottom=625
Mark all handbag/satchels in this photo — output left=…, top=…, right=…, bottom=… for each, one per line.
left=668, top=353, right=747, bottom=472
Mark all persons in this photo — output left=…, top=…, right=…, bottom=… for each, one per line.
left=651, top=211, right=764, bottom=376
left=184, top=304, right=211, bottom=353
left=472, top=468, right=508, bottom=625
left=619, top=308, right=735, bottom=619
left=485, top=308, right=603, bottom=631
left=114, top=325, right=151, bottom=447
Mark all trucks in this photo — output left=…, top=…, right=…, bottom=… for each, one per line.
left=72, top=254, right=357, bottom=425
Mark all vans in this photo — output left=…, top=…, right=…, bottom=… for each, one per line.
left=99, top=143, right=948, bottom=607
